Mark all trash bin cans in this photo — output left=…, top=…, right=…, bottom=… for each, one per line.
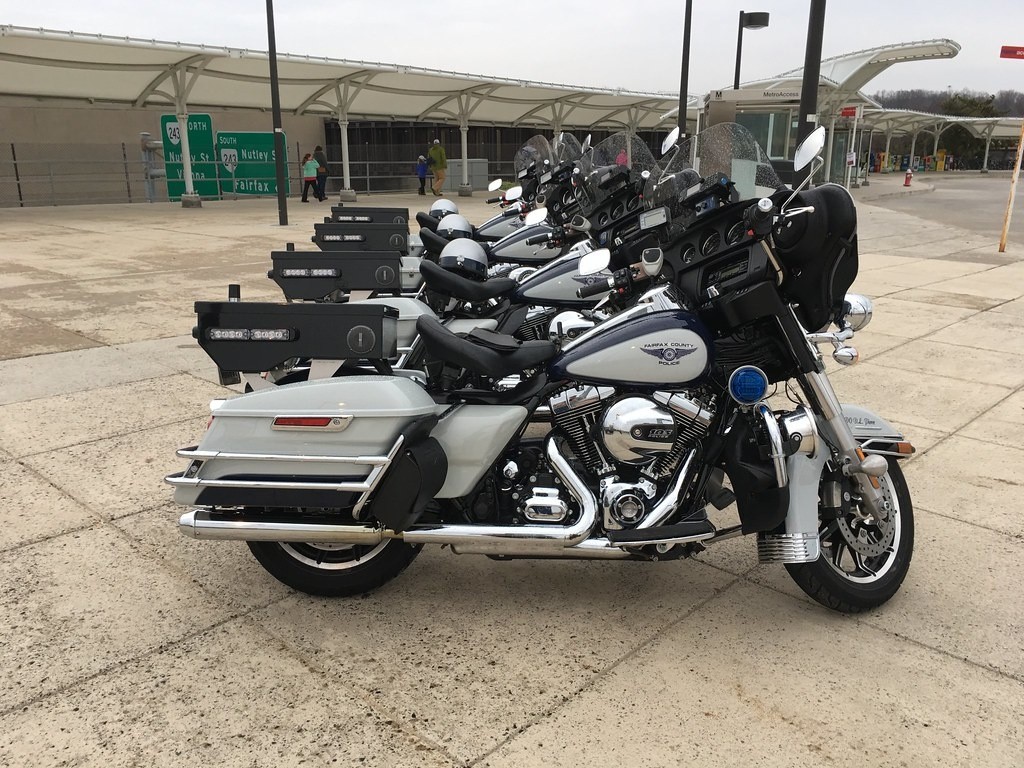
left=880, top=152, right=952, bottom=172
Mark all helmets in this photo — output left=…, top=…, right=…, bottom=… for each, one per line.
left=440, top=238, right=488, bottom=277
left=437, top=214, right=472, bottom=237
left=429, top=199, right=459, bottom=217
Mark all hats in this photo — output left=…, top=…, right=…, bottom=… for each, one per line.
left=434, top=139, right=439, bottom=144
left=419, top=155, right=426, bottom=160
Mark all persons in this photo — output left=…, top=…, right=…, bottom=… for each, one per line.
left=415, top=138, right=448, bottom=196
left=299, top=144, right=332, bottom=203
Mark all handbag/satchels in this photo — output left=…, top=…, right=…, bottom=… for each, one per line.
left=318, top=166, right=326, bottom=172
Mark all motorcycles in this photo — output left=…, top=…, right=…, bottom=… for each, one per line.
left=162, top=122, right=917, bottom=616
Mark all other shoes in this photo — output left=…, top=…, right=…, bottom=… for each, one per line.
left=431, top=187, right=436, bottom=195
left=427, top=156, right=435, bottom=166
left=302, top=199, right=309, bottom=202
left=418, top=190, right=426, bottom=195
left=319, top=196, right=328, bottom=202
left=435, top=194, right=443, bottom=196
